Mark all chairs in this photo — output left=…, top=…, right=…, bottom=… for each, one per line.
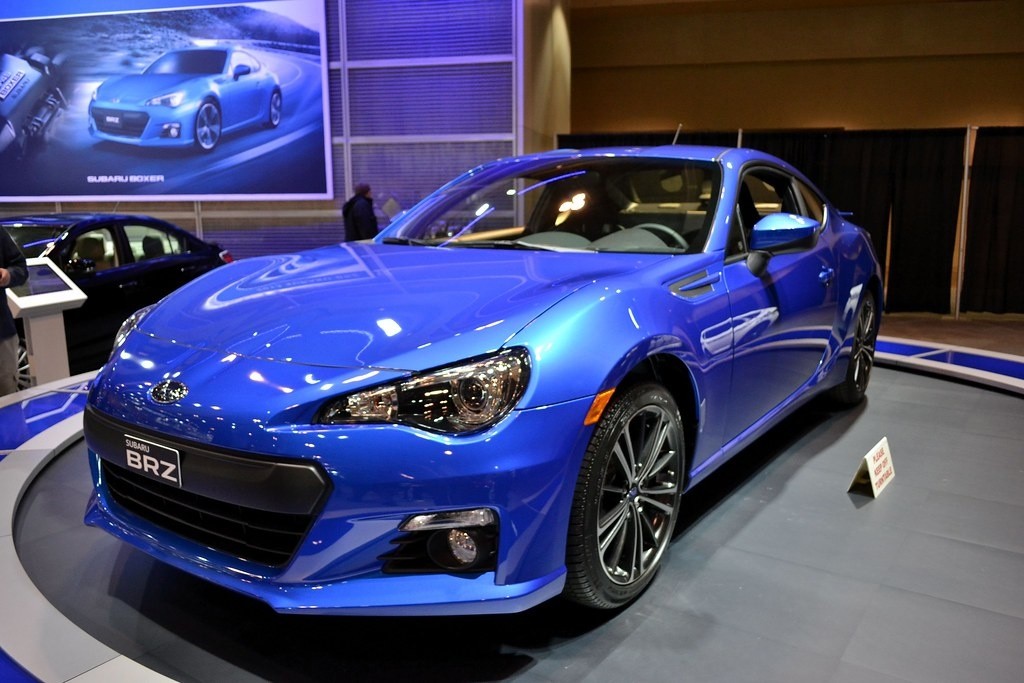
left=138, top=236, right=165, bottom=264
left=72, top=238, right=112, bottom=273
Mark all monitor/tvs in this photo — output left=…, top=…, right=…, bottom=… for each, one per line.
left=9, top=264, right=72, bottom=297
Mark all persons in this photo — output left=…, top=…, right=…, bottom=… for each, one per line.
left=0, top=221, right=29, bottom=397
left=342, top=183, right=378, bottom=242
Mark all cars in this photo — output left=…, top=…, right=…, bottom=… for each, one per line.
left=0, top=212, right=236, bottom=395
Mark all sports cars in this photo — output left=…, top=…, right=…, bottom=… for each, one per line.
left=83, top=142, right=884, bottom=619
left=88, top=45, right=283, bottom=150
